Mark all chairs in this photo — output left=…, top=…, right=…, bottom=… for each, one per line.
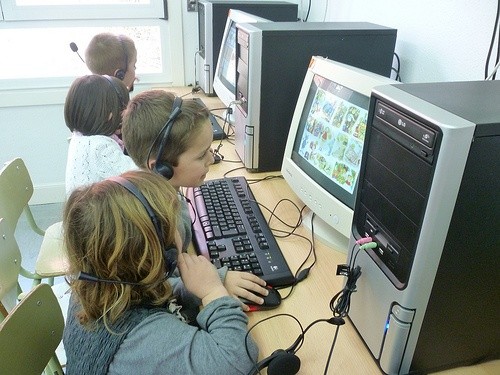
left=0, top=157, right=69, bottom=375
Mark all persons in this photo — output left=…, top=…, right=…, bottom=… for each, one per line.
left=122, top=91, right=269, bottom=311
left=64, top=172, right=258, bottom=375
left=85, top=32, right=137, bottom=86
left=64, top=75, right=137, bottom=197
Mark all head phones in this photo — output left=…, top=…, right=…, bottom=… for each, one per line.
left=103, top=174, right=179, bottom=287
left=103, top=74, right=127, bottom=129
left=247, top=349, right=300, bottom=375
left=114, top=37, right=128, bottom=80
left=153, top=96, right=184, bottom=181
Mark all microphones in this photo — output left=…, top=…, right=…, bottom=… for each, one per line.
left=70, top=42, right=86, bottom=63
left=287, top=316, right=346, bottom=353
left=77, top=271, right=140, bottom=286
left=147, top=107, right=182, bottom=171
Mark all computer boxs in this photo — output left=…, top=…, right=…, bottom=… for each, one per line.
left=342, top=79, right=500, bottom=375
left=235, top=22, right=398, bottom=173
left=196, top=0, right=299, bottom=97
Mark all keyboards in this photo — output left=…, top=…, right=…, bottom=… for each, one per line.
left=186, top=176, right=295, bottom=285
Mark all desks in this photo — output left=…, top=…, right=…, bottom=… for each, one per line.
left=152, top=85, right=500, bottom=375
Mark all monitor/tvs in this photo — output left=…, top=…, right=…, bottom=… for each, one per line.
left=280, top=55, right=404, bottom=254
left=213, top=10, right=273, bottom=124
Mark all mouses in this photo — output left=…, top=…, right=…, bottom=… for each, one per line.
left=237, top=284, right=282, bottom=311
